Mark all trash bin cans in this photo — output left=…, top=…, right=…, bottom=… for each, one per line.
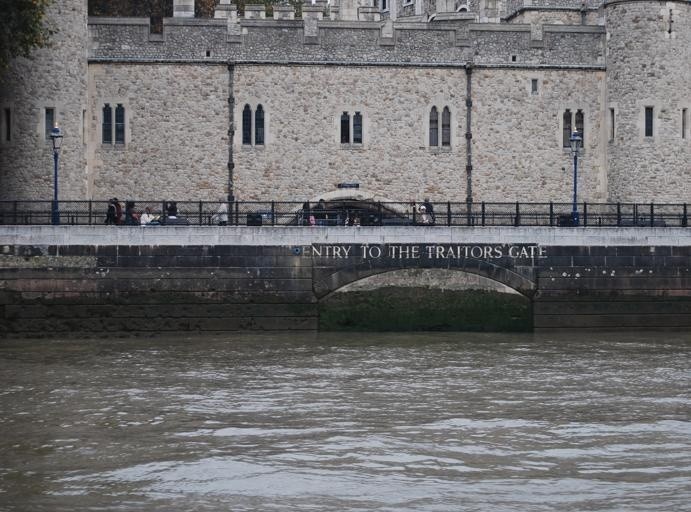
left=246, top=214, right=262, bottom=226
left=558, top=214, right=574, bottom=227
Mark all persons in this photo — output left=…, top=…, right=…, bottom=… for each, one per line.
left=416, top=198, right=435, bottom=225
left=104, top=197, right=177, bottom=226
left=298, top=199, right=356, bottom=227
left=210, top=197, right=228, bottom=226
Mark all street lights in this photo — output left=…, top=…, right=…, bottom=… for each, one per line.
left=50, top=121, right=64, bottom=226
left=568, top=127, right=582, bottom=227
